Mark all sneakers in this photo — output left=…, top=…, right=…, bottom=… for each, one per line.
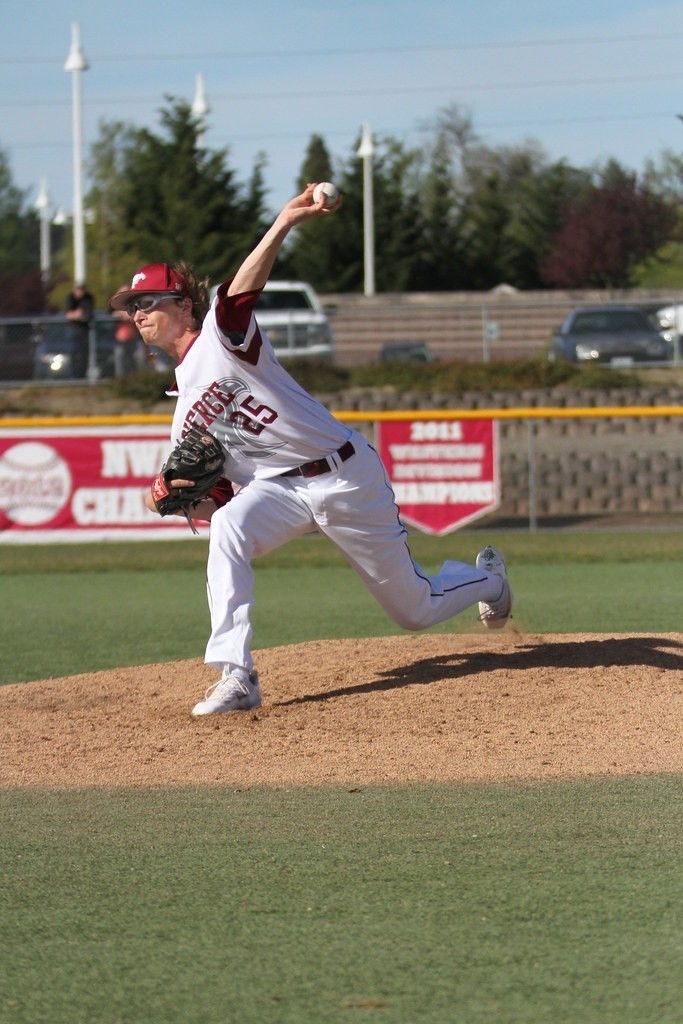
left=476, top=546, right=512, bottom=631
left=192, top=669, right=261, bottom=717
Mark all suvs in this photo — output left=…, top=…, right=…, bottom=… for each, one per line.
left=212, top=281, right=339, bottom=384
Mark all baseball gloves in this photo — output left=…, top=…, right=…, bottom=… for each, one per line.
left=151, top=424, right=226, bottom=519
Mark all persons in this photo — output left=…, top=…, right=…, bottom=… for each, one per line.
left=109, top=182, right=513, bottom=717
left=64, top=282, right=142, bottom=378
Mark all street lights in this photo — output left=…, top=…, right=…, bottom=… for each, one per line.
left=65, top=23, right=91, bottom=284
left=34, top=173, right=53, bottom=289
left=357, top=126, right=377, bottom=300
left=186, top=70, right=209, bottom=154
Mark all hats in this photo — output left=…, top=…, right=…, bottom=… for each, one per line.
left=108, top=262, right=192, bottom=310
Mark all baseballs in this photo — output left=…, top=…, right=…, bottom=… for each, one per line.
left=313, top=182, right=338, bottom=208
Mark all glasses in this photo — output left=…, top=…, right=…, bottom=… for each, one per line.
left=125, top=294, right=184, bottom=318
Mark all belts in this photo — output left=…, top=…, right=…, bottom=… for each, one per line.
left=280, top=441, right=356, bottom=478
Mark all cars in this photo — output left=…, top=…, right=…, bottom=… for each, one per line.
left=547, top=303, right=683, bottom=368
left=1, top=311, right=174, bottom=381
